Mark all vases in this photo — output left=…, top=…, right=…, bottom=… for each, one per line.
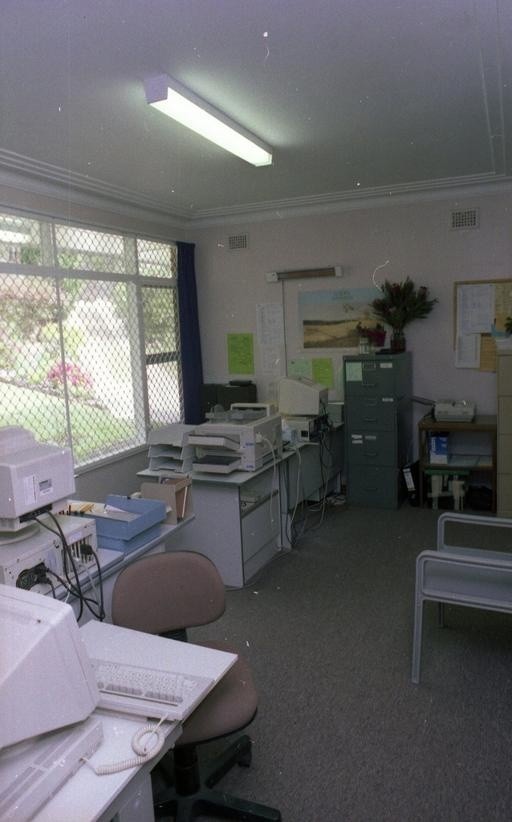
left=391, top=332, right=406, bottom=353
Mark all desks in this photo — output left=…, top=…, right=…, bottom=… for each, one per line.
left=138, top=421, right=345, bottom=589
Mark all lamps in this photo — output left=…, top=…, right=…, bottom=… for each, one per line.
left=142, top=73, right=273, bottom=168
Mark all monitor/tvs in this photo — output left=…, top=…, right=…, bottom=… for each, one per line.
left=0, top=586, right=101, bottom=761
left=0, top=425, right=76, bottom=545
left=276, top=374, right=328, bottom=419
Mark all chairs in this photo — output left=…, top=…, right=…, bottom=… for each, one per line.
left=110, top=549, right=281, bottom=821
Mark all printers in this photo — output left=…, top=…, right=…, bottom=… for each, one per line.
left=185, top=402, right=282, bottom=474
left=408, top=395, right=476, bottom=422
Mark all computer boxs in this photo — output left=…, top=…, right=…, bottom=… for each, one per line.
left=279, top=417, right=330, bottom=442
left=0, top=515, right=98, bottom=592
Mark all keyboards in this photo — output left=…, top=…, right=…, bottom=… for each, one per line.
left=95, top=658, right=214, bottom=720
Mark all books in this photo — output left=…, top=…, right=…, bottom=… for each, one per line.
left=191, top=453, right=243, bottom=475
left=188, top=432, right=240, bottom=452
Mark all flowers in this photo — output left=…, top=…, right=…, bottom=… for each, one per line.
left=366, top=274, right=440, bottom=349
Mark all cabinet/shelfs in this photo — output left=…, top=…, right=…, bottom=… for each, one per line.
left=342, top=352, right=413, bottom=511
left=238, top=462, right=283, bottom=586
left=416, top=413, right=497, bottom=514
